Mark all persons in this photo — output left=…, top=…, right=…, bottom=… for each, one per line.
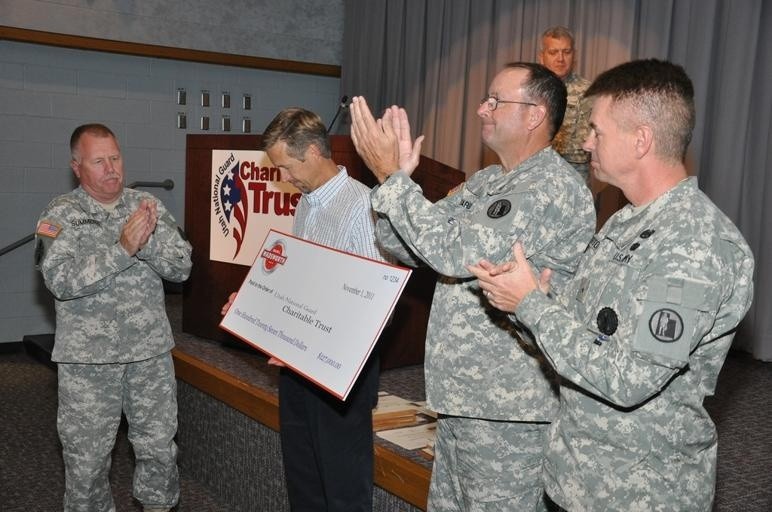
left=464, top=57, right=757, bottom=512
left=534, top=24, right=605, bottom=201
left=34, top=123, right=195, bottom=512
left=349, top=60, right=601, bottom=512
left=214, top=107, right=411, bottom=511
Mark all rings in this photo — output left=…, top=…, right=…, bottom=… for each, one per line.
left=485, top=291, right=490, bottom=299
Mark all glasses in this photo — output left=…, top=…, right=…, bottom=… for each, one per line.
left=480, top=97, right=537, bottom=111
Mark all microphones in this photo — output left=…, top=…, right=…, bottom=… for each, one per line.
left=327, top=95, right=348, bottom=133
